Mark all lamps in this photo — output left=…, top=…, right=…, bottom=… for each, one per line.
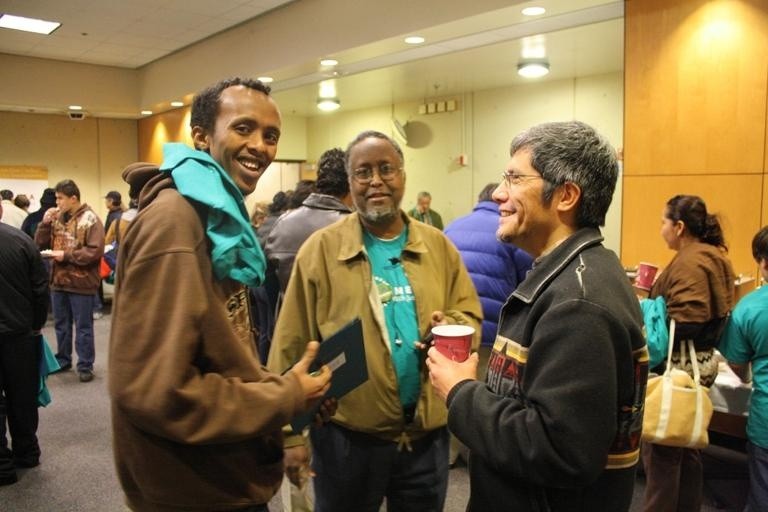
left=317, top=98, right=340, bottom=112
left=517, top=63, right=548, bottom=79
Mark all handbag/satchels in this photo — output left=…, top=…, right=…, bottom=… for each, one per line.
left=640, top=369, right=713, bottom=450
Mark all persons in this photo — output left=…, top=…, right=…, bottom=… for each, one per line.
left=106, top=76, right=333, bottom=512
left=0, top=194, right=51, bottom=488
left=1, top=188, right=140, bottom=328
left=636, top=194, right=735, bottom=511
left=442, top=184, right=535, bottom=471
left=426, top=120, right=650, bottom=512
left=266, top=131, right=485, bottom=512
left=251, top=149, right=356, bottom=360
left=34, top=179, right=106, bottom=383
left=411, top=192, right=443, bottom=231
left=716, top=225, right=768, bottom=512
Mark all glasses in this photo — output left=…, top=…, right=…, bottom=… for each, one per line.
left=502, top=170, right=542, bottom=190
left=350, top=164, right=402, bottom=185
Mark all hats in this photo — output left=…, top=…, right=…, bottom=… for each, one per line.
left=106, top=191, right=121, bottom=202
left=41, top=189, right=56, bottom=205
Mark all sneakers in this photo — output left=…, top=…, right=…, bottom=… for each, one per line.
left=0, top=458, right=39, bottom=485
left=80, top=371, right=92, bottom=382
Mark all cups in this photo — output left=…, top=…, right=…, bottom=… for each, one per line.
left=431, top=325, right=476, bottom=363
left=637, top=264, right=658, bottom=291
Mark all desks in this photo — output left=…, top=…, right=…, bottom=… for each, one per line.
left=639, top=362, right=753, bottom=508
